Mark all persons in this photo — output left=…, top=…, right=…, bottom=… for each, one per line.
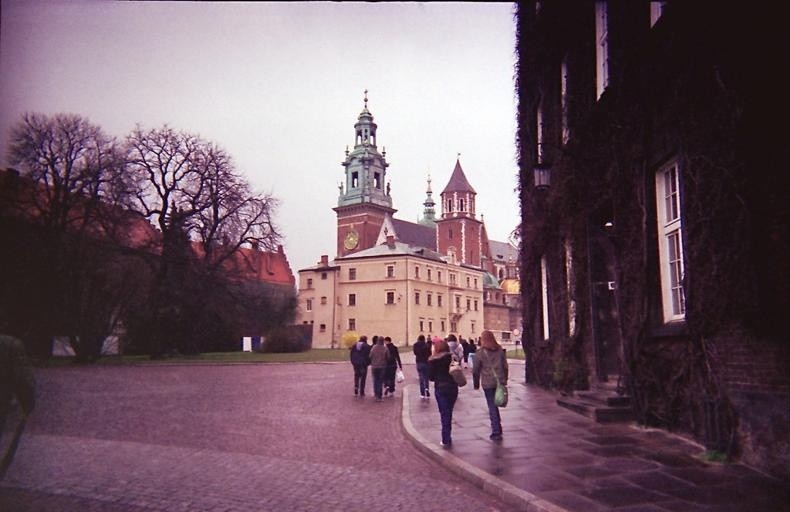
left=413, top=335, right=432, bottom=398
left=472, top=330, right=509, bottom=441
left=426, top=334, right=481, bottom=368
left=428, top=340, right=458, bottom=447
left=349, top=334, right=403, bottom=401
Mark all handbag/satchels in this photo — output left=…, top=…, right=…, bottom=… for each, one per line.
left=494, top=384, right=508, bottom=407
left=448, top=360, right=466, bottom=387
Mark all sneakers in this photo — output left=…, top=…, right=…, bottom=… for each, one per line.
left=490, top=431, right=502, bottom=439
left=425, top=388, right=430, bottom=397
left=354, top=386, right=396, bottom=402
